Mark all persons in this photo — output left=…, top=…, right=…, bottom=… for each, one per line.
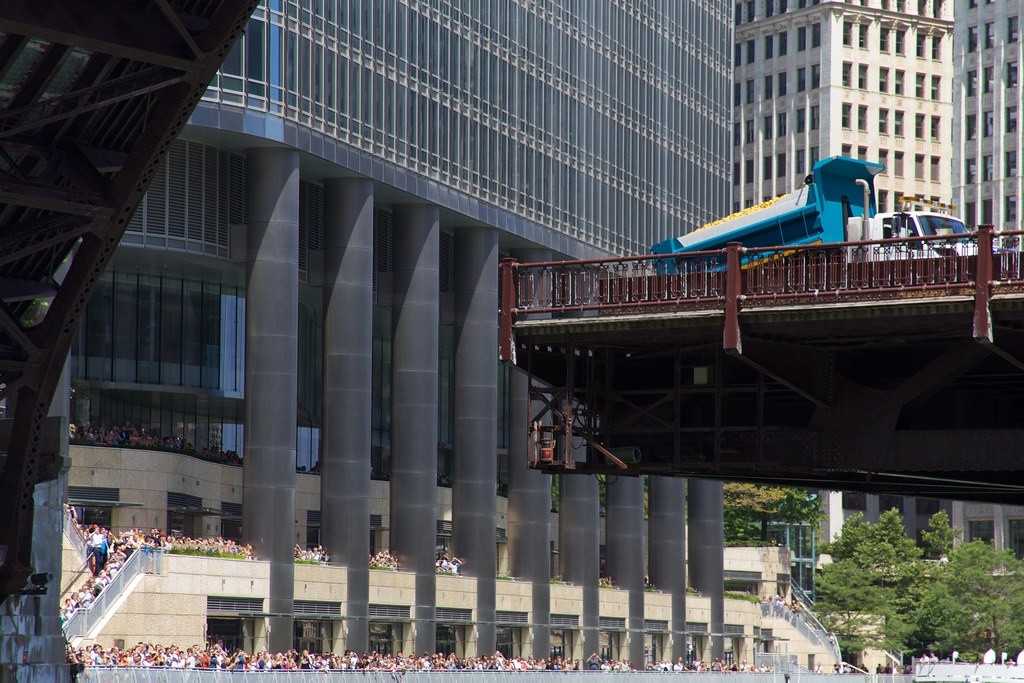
left=0, top=417, right=1024, bottom=682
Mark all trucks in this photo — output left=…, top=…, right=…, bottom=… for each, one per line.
left=648, top=155, right=1014, bottom=275
